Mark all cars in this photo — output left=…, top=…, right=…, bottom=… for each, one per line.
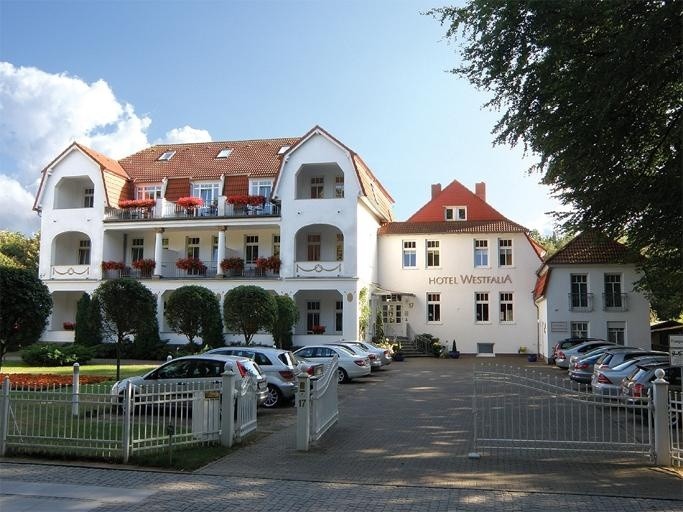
left=554, top=338, right=683, bottom=429
left=175, top=341, right=394, bottom=409
left=111, top=354, right=270, bottom=418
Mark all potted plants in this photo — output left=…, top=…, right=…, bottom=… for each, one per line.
left=117, top=198, right=156, bottom=219
left=248, top=196, right=264, bottom=211
left=419, top=333, right=460, bottom=359
left=312, top=324, right=325, bottom=334
left=175, top=257, right=205, bottom=274
left=132, top=258, right=155, bottom=277
left=220, top=255, right=282, bottom=278
left=102, top=261, right=124, bottom=279
left=391, top=341, right=404, bottom=361
left=225, top=195, right=248, bottom=211
left=64, top=321, right=75, bottom=330
left=176, top=196, right=203, bottom=214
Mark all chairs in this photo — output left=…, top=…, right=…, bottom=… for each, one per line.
left=207, top=366, right=216, bottom=377
left=194, top=365, right=207, bottom=377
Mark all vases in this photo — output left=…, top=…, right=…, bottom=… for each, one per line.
left=527, top=354, right=537, bottom=362
